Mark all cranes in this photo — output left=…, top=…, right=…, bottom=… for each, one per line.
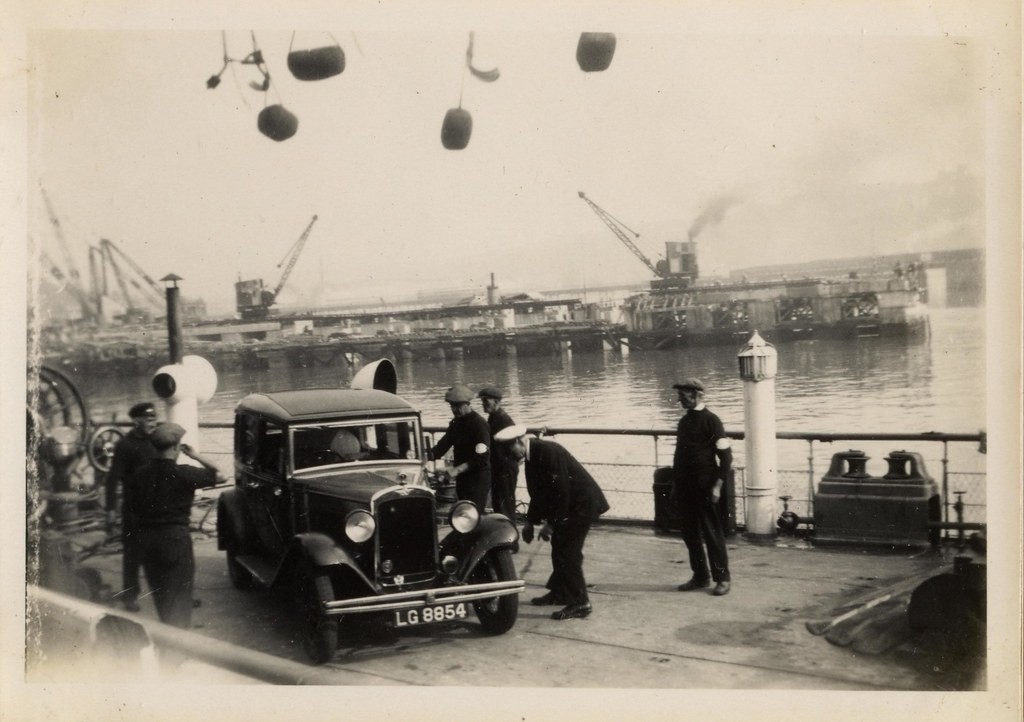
left=234, top=213, right=321, bottom=318
left=580, top=190, right=699, bottom=293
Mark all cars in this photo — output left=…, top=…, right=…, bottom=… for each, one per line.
left=217, top=390, right=524, bottom=665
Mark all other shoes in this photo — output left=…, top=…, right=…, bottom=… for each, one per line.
left=124, top=598, right=140, bottom=611
left=191, top=599, right=200, bottom=606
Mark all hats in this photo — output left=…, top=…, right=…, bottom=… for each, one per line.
left=445, top=385, right=473, bottom=402
left=493, top=425, right=526, bottom=448
left=672, top=378, right=704, bottom=391
left=128, top=402, right=156, bottom=418
left=476, top=389, right=501, bottom=401
left=151, top=422, right=186, bottom=447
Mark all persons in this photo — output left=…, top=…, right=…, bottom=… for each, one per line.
left=105, top=402, right=199, bottom=611
left=423, top=386, right=491, bottom=514
left=476, top=388, right=523, bottom=553
left=494, top=424, right=608, bottom=620
left=671, top=377, right=732, bottom=596
left=129, top=423, right=228, bottom=631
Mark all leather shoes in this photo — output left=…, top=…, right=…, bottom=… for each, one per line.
left=552, top=601, right=592, bottom=619
left=713, top=581, right=730, bottom=595
left=532, top=592, right=566, bottom=605
left=679, top=578, right=710, bottom=590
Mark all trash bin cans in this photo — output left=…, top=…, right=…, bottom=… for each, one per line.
left=652, top=466, right=737, bottom=537
